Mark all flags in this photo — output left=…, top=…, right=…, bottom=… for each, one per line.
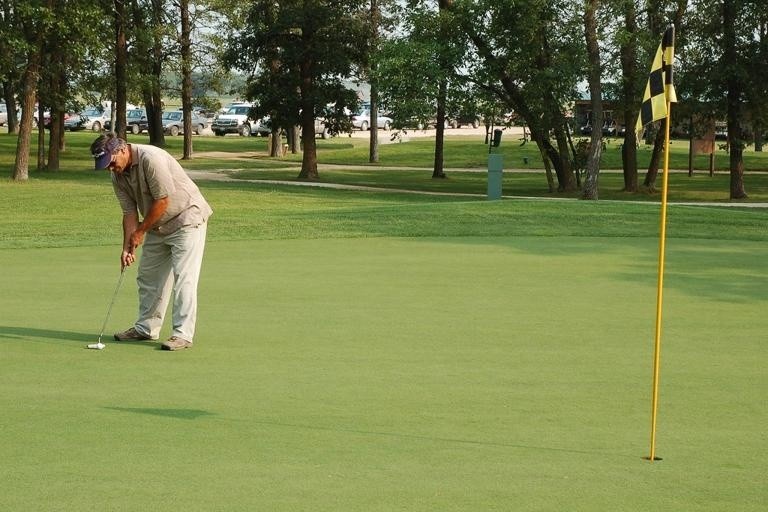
left=633, top=24, right=679, bottom=151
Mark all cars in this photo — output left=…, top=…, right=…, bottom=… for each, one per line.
left=0, top=101, right=209, bottom=136
left=282, top=110, right=331, bottom=140
left=351, top=108, right=528, bottom=131
left=580, top=118, right=626, bottom=136
left=714, top=126, right=747, bottom=140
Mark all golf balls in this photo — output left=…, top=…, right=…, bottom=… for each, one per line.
left=97, top=343, right=102, bottom=349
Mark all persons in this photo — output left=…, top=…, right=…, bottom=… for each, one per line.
left=89, top=132, right=214, bottom=352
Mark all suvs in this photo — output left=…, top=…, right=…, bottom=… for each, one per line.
left=211, top=102, right=271, bottom=137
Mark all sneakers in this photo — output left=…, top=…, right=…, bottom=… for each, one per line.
left=114, top=327, right=147, bottom=341
left=161, top=336, right=191, bottom=351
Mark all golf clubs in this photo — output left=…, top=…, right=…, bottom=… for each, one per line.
left=87, top=265, right=128, bottom=349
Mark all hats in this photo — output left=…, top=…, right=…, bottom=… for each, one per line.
left=91, top=134, right=118, bottom=171
